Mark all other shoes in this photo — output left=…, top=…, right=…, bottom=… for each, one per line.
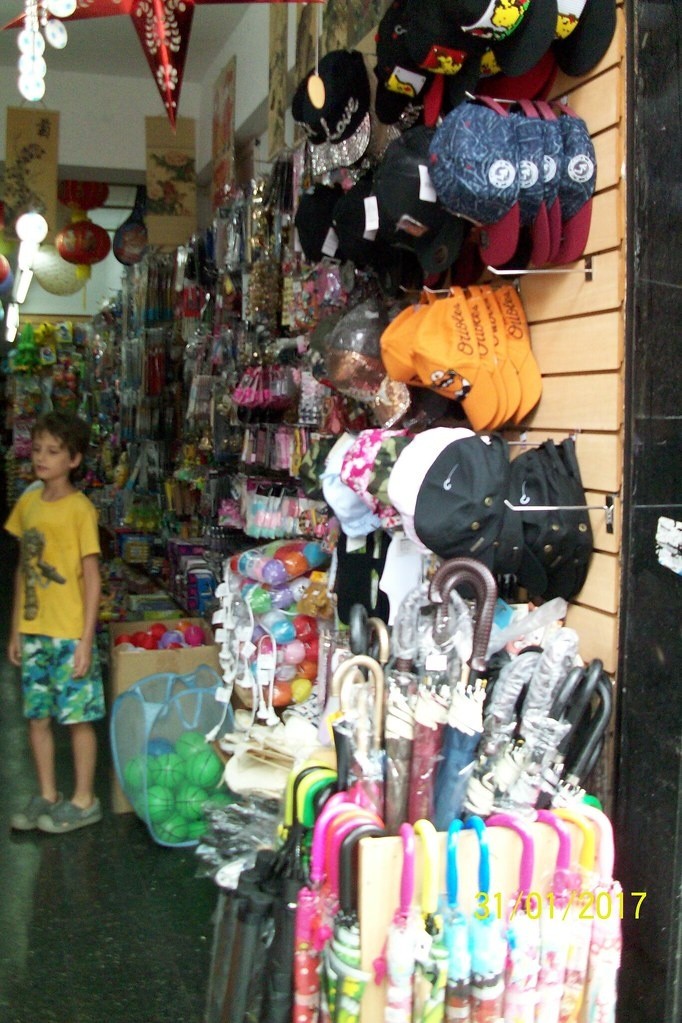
left=10, top=792, right=66, bottom=830
left=37, top=797, right=102, bottom=834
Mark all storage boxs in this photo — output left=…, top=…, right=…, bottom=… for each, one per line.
left=107, top=617, right=222, bottom=815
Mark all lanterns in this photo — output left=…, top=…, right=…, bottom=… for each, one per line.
left=57, top=180, right=111, bottom=283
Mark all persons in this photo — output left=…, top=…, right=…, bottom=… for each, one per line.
left=0, top=410, right=108, bottom=834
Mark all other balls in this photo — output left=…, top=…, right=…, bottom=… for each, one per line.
left=116, top=622, right=206, bottom=649
left=226, top=537, right=331, bottom=710
left=125, top=731, right=235, bottom=845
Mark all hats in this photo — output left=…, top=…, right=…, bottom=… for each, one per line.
left=289, top=0, right=617, bottom=607
